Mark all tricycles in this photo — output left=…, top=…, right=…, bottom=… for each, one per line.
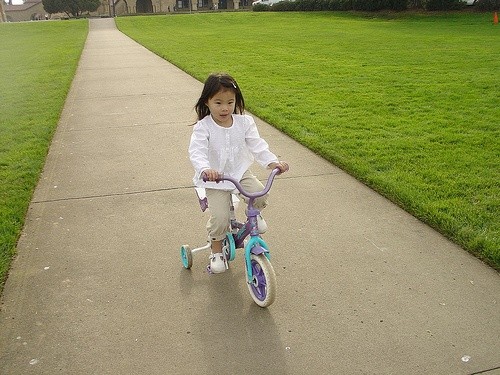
left=181, top=166, right=281, bottom=308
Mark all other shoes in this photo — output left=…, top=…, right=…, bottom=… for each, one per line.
left=210, top=250, right=226, bottom=273
left=245, top=206, right=268, bottom=234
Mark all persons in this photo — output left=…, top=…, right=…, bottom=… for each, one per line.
left=190, top=74, right=289, bottom=274
